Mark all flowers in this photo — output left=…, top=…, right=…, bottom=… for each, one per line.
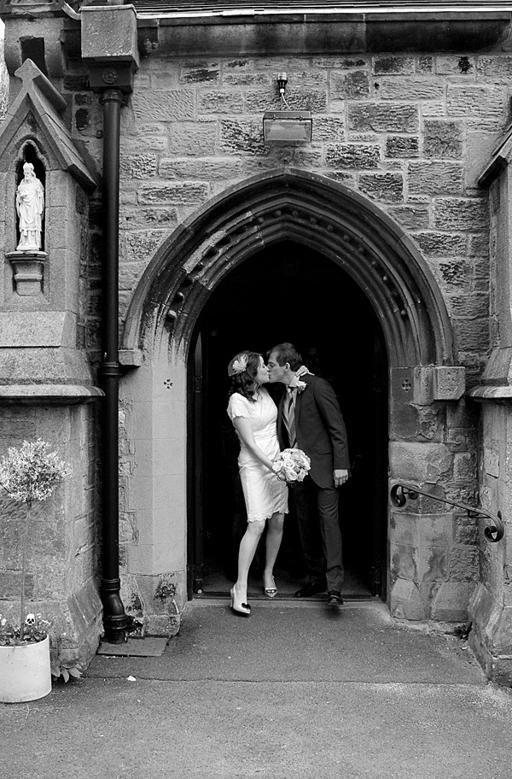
left=272, top=447, right=313, bottom=484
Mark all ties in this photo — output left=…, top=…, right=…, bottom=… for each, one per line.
left=285, top=387, right=297, bottom=447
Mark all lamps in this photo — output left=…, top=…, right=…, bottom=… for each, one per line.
left=259, top=69, right=316, bottom=143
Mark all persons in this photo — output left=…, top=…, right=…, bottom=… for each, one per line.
left=225, top=348, right=290, bottom=614
left=267, top=343, right=351, bottom=608
left=14, top=162, right=46, bottom=251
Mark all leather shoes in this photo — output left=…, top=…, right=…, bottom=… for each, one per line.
left=327, top=591, right=342, bottom=614
left=265, top=576, right=278, bottom=597
left=294, top=582, right=326, bottom=598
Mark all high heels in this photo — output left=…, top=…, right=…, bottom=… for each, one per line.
left=229, top=586, right=251, bottom=614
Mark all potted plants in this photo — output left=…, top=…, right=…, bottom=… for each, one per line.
left=0, top=433, right=65, bottom=707
